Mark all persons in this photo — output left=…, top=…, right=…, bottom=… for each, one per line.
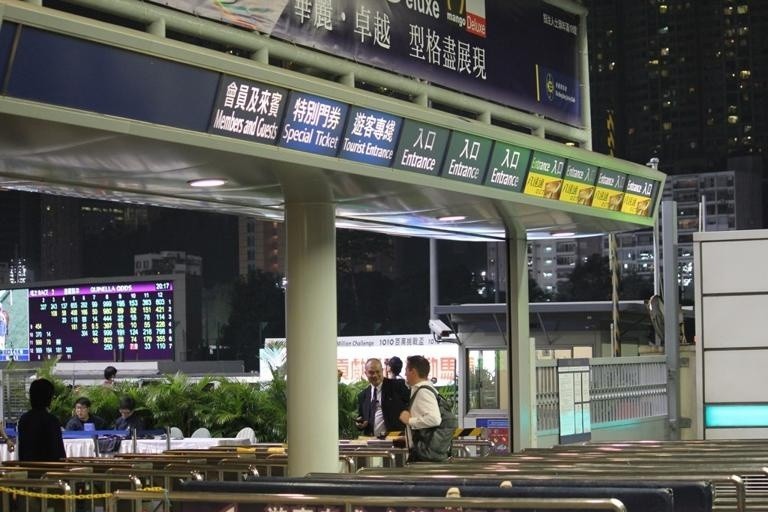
left=65, top=396, right=109, bottom=432
left=113, top=396, right=139, bottom=430
left=338, top=370, right=343, bottom=382
left=384, top=355, right=403, bottom=379
left=399, top=355, right=442, bottom=462
left=17, top=377, right=75, bottom=511
left=356, top=358, right=409, bottom=437
left=101, top=366, right=117, bottom=385
left=0, top=420, right=15, bottom=453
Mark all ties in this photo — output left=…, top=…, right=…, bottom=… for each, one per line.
left=366, top=386, right=376, bottom=436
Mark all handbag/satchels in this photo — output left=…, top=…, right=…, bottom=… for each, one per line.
left=126, top=407, right=155, bottom=431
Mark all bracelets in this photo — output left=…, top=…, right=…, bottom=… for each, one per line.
left=4, top=438, right=10, bottom=443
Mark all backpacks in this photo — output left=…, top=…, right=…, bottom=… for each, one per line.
left=408, top=385, right=457, bottom=462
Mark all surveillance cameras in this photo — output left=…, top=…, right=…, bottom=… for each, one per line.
left=428, top=318, right=452, bottom=342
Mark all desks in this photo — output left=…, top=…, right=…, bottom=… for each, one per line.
left=1, top=438, right=251, bottom=462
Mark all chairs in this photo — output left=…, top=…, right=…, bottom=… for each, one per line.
left=168, top=427, right=256, bottom=446
